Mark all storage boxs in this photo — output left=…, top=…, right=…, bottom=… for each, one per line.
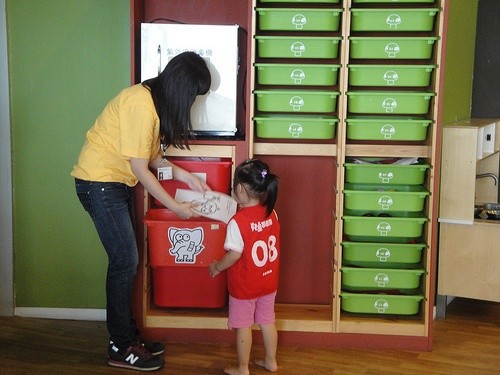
left=141, top=6, right=443, bottom=317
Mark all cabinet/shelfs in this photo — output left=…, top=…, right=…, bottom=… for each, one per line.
left=129, top=0, right=450, bottom=351
left=439, top=119, right=500, bottom=224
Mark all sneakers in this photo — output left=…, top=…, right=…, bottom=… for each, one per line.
left=107, top=336, right=166, bottom=372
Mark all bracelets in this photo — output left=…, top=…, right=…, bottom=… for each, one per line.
left=214, top=264, right=221, bottom=272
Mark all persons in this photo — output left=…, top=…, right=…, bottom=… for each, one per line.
left=71, top=50, right=211, bottom=371
left=208, top=161, right=280, bottom=375
